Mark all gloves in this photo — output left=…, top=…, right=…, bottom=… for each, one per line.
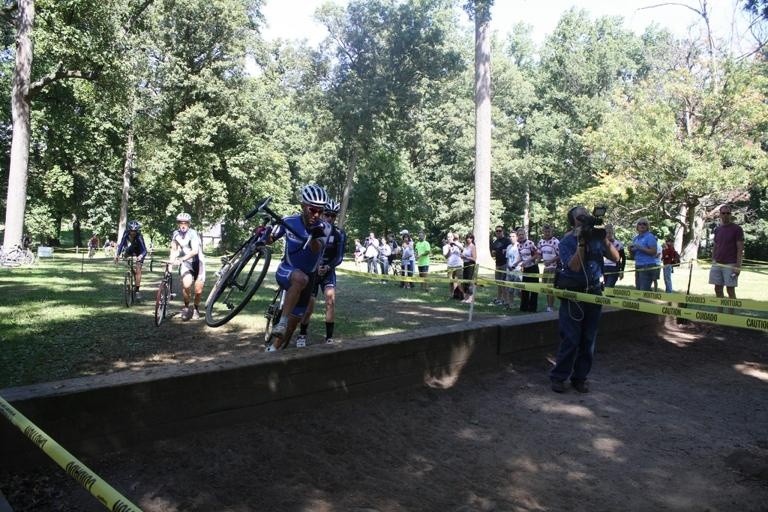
left=254, top=226, right=267, bottom=237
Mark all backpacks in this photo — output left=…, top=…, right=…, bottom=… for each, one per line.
left=671, top=248, right=680, bottom=267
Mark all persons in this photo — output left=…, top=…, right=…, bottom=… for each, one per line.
left=296, top=198, right=346, bottom=348
left=113, top=221, right=147, bottom=299
left=442, top=232, right=477, bottom=304
left=549, top=207, right=620, bottom=393
left=708, top=206, right=745, bottom=314
left=353, top=229, right=431, bottom=289
left=256, top=185, right=333, bottom=352
left=492, top=218, right=674, bottom=314
left=170, top=213, right=206, bottom=320
left=87, top=233, right=116, bottom=257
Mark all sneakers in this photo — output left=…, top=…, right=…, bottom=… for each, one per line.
left=551, top=378, right=567, bottom=394
left=192, top=306, right=200, bottom=320
left=264, top=347, right=271, bottom=352
left=546, top=305, right=553, bottom=313
left=324, top=338, right=335, bottom=344
left=460, top=297, right=475, bottom=304
left=271, top=315, right=289, bottom=341
left=181, top=306, right=193, bottom=321
left=487, top=297, right=512, bottom=314
left=295, top=334, right=307, bottom=348
left=570, top=377, right=591, bottom=394
left=400, top=282, right=415, bottom=289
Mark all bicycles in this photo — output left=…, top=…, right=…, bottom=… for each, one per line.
left=1, top=241, right=37, bottom=268
left=201, top=196, right=312, bottom=323
left=148, top=255, right=181, bottom=330
left=114, top=253, right=145, bottom=308
left=88, top=244, right=96, bottom=263
left=260, top=261, right=327, bottom=344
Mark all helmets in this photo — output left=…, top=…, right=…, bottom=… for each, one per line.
left=127, top=220, right=141, bottom=231
left=301, top=185, right=330, bottom=209
left=175, top=212, right=192, bottom=228
left=323, top=199, right=341, bottom=217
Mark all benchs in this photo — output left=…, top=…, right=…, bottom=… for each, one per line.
left=37, top=246, right=56, bottom=261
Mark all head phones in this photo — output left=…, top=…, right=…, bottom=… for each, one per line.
left=568, top=206, right=578, bottom=226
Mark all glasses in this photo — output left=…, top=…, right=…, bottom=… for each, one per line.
left=638, top=222, right=649, bottom=226
left=495, top=230, right=503, bottom=233
left=721, top=212, right=731, bottom=215
